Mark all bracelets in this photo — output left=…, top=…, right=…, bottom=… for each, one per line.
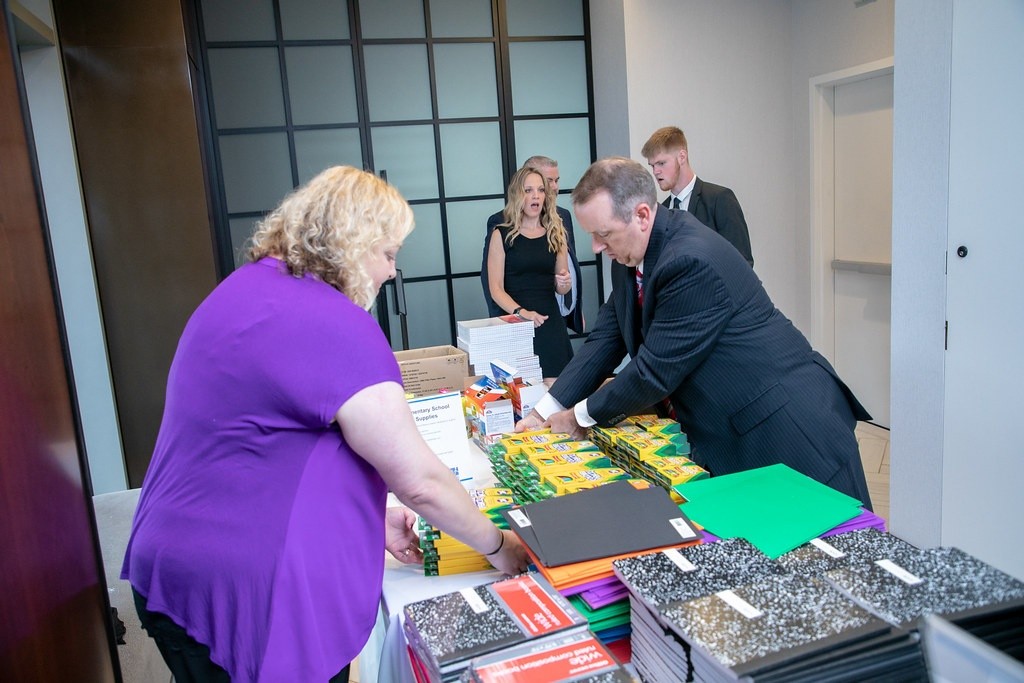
left=488, top=531, right=505, bottom=555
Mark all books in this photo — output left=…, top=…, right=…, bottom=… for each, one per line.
left=381, top=314, right=1024, bottom=683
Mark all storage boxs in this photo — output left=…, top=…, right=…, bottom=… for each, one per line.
left=490, top=359, right=548, bottom=414
left=464, top=376, right=515, bottom=450
left=392, top=345, right=468, bottom=398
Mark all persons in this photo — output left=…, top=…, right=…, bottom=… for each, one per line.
left=514, top=156, right=874, bottom=510
left=487, top=168, right=574, bottom=378
left=117, top=164, right=530, bottom=683
left=641, top=126, right=754, bottom=269
left=480, top=155, right=583, bottom=336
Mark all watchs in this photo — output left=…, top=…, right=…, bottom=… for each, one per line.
left=513, top=307, right=525, bottom=314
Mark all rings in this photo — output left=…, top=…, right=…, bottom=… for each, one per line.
left=406, top=549, right=410, bottom=555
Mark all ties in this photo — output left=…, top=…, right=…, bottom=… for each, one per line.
left=636, top=268, right=643, bottom=307
left=564, top=270, right=572, bottom=311
left=673, top=198, right=681, bottom=209
